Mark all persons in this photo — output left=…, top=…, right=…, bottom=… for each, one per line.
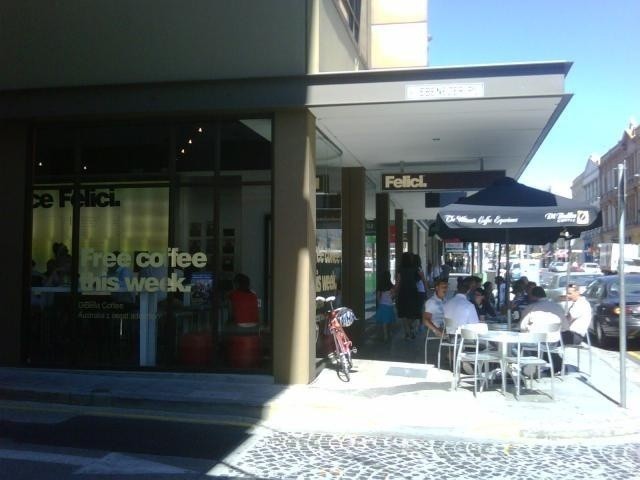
left=31, top=242, right=136, bottom=314
left=375, top=251, right=593, bottom=375
left=223, top=272, right=260, bottom=337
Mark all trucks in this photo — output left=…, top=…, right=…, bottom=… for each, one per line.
left=598, top=242, right=640, bottom=275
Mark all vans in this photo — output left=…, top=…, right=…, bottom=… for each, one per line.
left=542, top=272, right=602, bottom=315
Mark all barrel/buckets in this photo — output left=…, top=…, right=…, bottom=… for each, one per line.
left=226, top=334, right=263, bottom=373
left=179, top=330, right=212, bottom=373
left=463, top=323, right=488, bottom=340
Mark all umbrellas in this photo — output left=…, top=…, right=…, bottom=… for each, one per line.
left=428, top=176, right=603, bottom=321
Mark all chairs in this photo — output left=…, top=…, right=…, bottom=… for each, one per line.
left=422, top=311, right=594, bottom=401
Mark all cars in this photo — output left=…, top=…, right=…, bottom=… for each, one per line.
left=363, top=245, right=397, bottom=286
left=482, top=248, right=570, bottom=286
left=623, top=258, right=640, bottom=275
left=580, top=262, right=604, bottom=275
left=580, top=274, right=640, bottom=347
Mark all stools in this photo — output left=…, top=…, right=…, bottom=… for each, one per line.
left=182, top=331, right=215, bottom=367
left=224, top=332, right=260, bottom=373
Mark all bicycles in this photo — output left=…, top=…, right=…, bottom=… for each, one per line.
left=316, top=295, right=358, bottom=382
